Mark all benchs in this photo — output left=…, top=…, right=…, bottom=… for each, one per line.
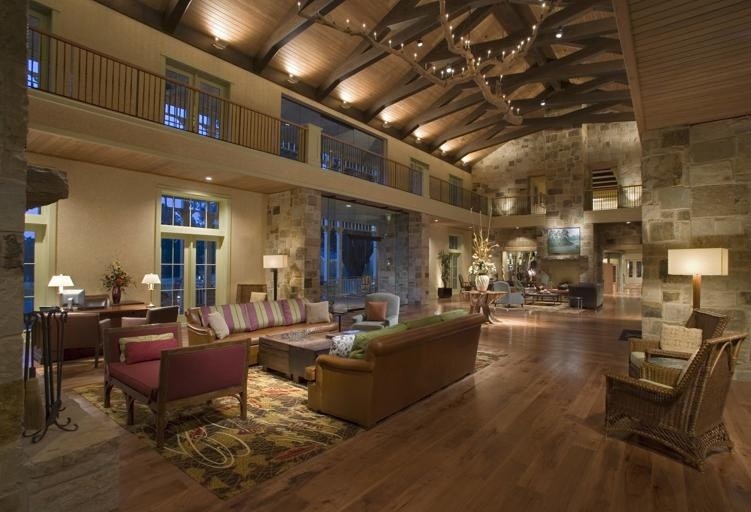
left=102, top=323, right=253, bottom=443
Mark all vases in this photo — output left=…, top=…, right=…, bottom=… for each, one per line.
left=112, top=284, right=121, bottom=304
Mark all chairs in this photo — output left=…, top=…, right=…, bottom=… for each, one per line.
left=628, top=308, right=730, bottom=384
left=75, top=294, right=110, bottom=309
left=121, top=306, right=179, bottom=326
left=250, top=292, right=268, bottom=302
left=61, top=312, right=111, bottom=369
left=458, top=271, right=585, bottom=307
left=352, top=292, right=401, bottom=330
left=604, top=332, right=750, bottom=475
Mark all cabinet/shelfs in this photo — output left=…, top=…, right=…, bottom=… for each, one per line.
left=237, top=283, right=270, bottom=303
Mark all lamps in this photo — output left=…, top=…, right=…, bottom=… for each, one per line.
left=263, top=255, right=289, bottom=300
left=140, top=273, right=162, bottom=307
left=48, top=273, right=74, bottom=294
left=667, top=247, right=731, bottom=310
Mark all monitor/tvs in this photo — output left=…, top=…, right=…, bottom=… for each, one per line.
left=60, top=288, right=85, bottom=311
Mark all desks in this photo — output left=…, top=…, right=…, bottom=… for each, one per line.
left=32, top=303, right=150, bottom=364
left=461, top=291, right=510, bottom=325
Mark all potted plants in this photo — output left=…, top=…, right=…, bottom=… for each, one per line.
left=436, top=249, right=454, bottom=298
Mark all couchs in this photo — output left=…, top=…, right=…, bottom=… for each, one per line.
left=303, top=308, right=486, bottom=430
left=185, top=296, right=339, bottom=367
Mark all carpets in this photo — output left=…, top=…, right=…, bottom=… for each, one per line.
left=69, top=346, right=511, bottom=502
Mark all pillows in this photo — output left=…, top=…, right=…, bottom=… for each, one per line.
left=125, top=338, right=177, bottom=364
left=207, top=311, right=230, bottom=340
left=118, top=332, right=175, bottom=362
left=368, top=301, right=388, bottom=321
left=329, top=334, right=357, bottom=358
left=305, top=300, right=332, bottom=324
left=660, top=322, right=704, bottom=355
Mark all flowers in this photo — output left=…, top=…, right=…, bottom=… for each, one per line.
left=464, top=205, right=501, bottom=275
left=98, top=257, right=138, bottom=296
